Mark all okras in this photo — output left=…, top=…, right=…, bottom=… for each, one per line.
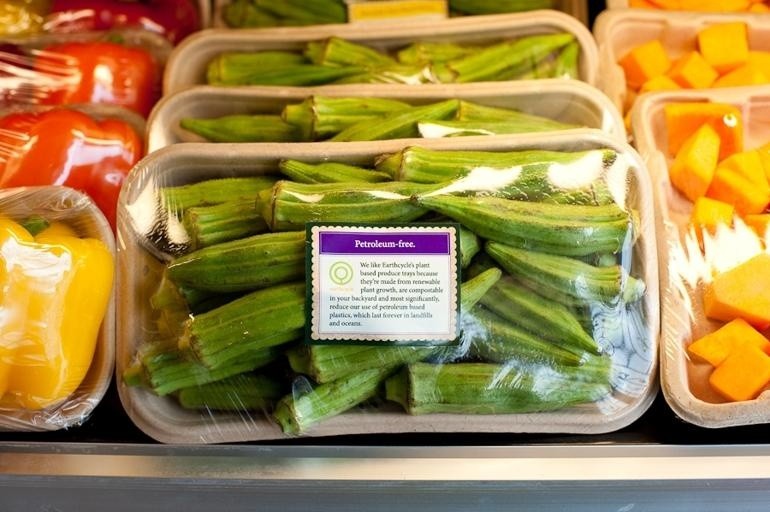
left=123, top=0, right=645, bottom=438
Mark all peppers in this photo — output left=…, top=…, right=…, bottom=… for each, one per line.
left=0, top=0, right=201, bottom=412
